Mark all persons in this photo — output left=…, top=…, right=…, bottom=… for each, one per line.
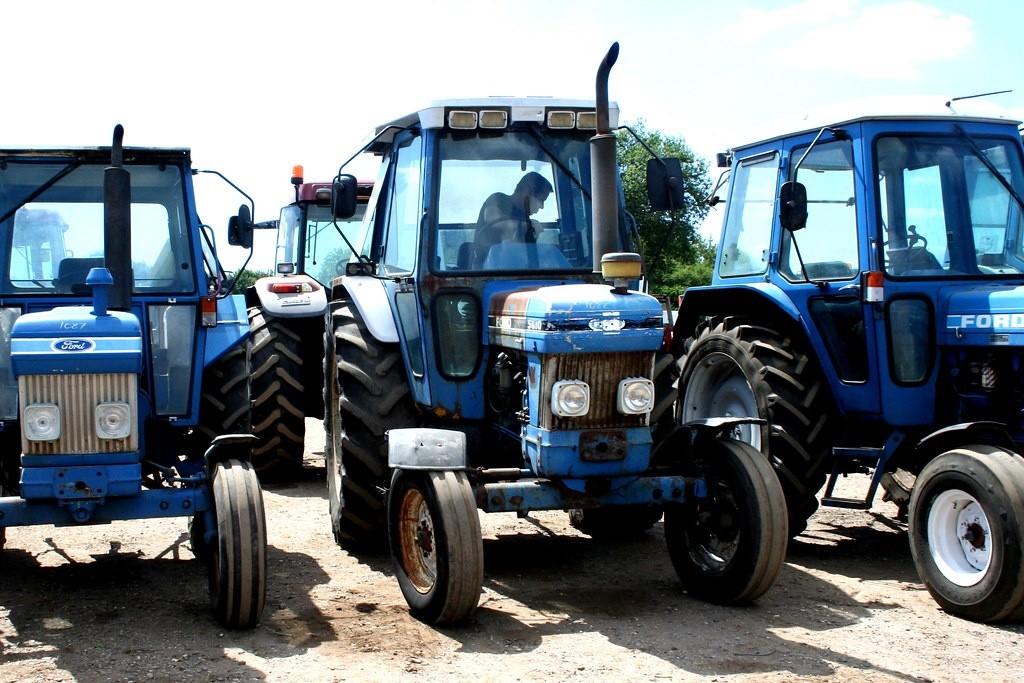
left=474, top=172, right=554, bottom=264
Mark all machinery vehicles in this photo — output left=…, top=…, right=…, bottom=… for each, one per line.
left=0, top=124, right=267, bottom=630
left=227, top=42, right=789, bottom=631
left=648, top=89, right=1024, bottom=626
